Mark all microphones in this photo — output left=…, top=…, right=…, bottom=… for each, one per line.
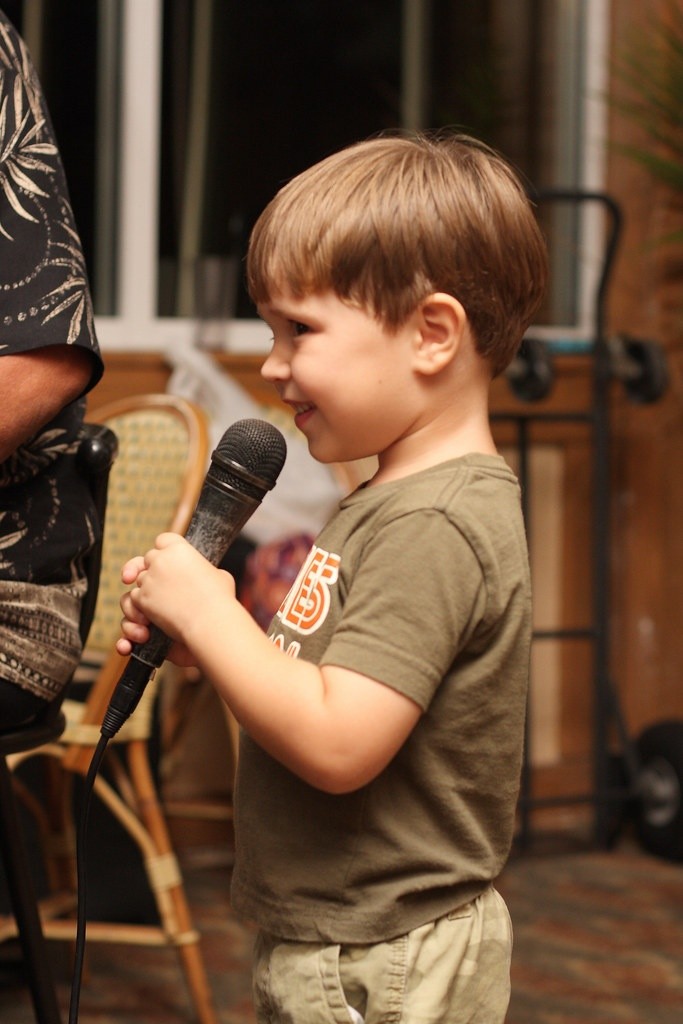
left=101, top=418, right=287, bottom=738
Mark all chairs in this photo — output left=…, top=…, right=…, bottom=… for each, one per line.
left=0, top=395, right=211, bottom=1024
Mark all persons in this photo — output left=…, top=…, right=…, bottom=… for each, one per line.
left=0, top=9, right=106, bottom=735
left=116, top=134, right=550, bottom=1024
